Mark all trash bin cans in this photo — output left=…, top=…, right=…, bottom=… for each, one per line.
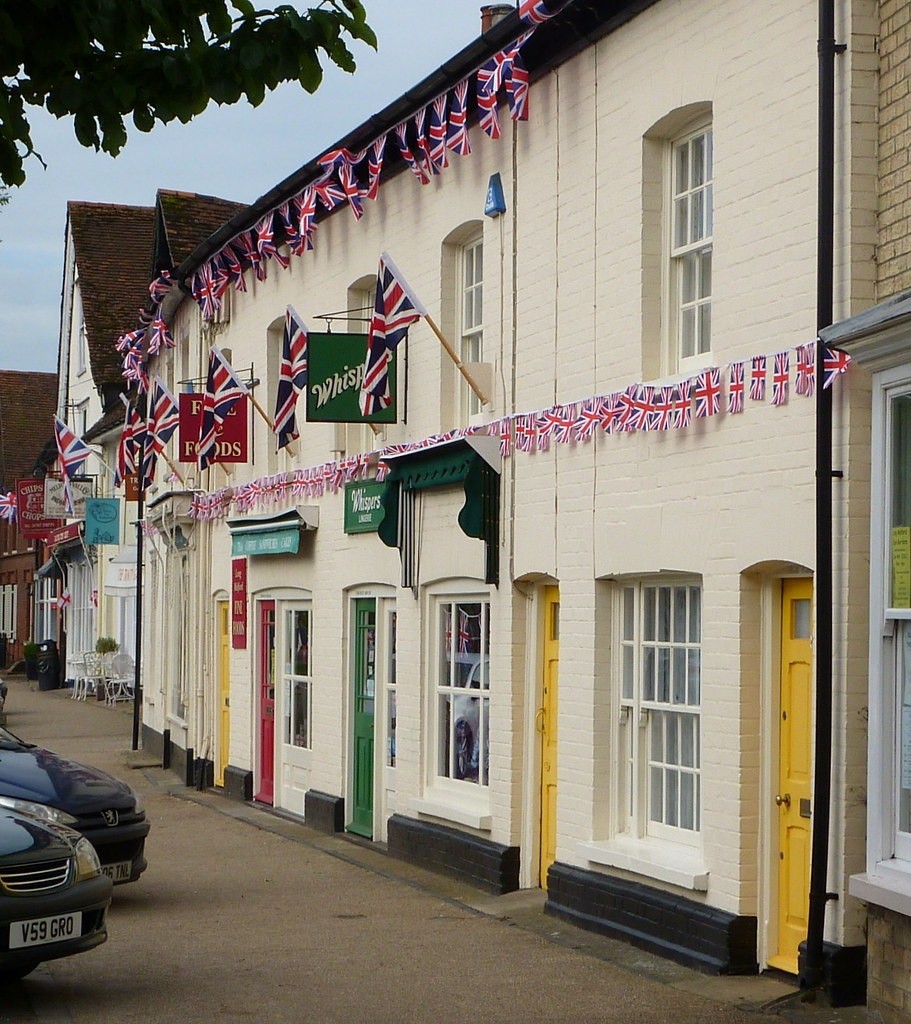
left=0, top=633, right=7, bottom=668
left=36, top=640, right=60, bottom=691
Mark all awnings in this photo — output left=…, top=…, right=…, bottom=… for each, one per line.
left=229, top=518, right=305, bottom=555
left=104, top=545, right=137, bottom=596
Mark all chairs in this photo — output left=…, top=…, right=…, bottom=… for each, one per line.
left=69, top=651, right=134, bottom=708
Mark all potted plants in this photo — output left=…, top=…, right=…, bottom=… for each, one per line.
left=24, top=644, right=40, bottom=680
left=93, top=636, right=120, bottom=701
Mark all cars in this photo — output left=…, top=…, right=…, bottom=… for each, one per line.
left=0, top=725, right=150, bottom=886
left=0, top=805, right=114, bottom=980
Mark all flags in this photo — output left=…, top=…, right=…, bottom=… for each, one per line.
left=358, top=258, right=420, bottom=418
left=188, top=340, right=851, bottom=520
left=57, top=589, right=71, bottom=610
left=272, top=310, right=307, bottom=454
left=54, top=418, right=91, bottom=517
left=446, top=612, right=470, bottom=657
left=115, top=1, right=564, bottom=381
left=198, top=351, right=243, bottom=471
left=0, top=489, right=17, bottom=524
left=142, top=379, right=179, bottom=491
left=114, top=403, right=146, bottom=488
